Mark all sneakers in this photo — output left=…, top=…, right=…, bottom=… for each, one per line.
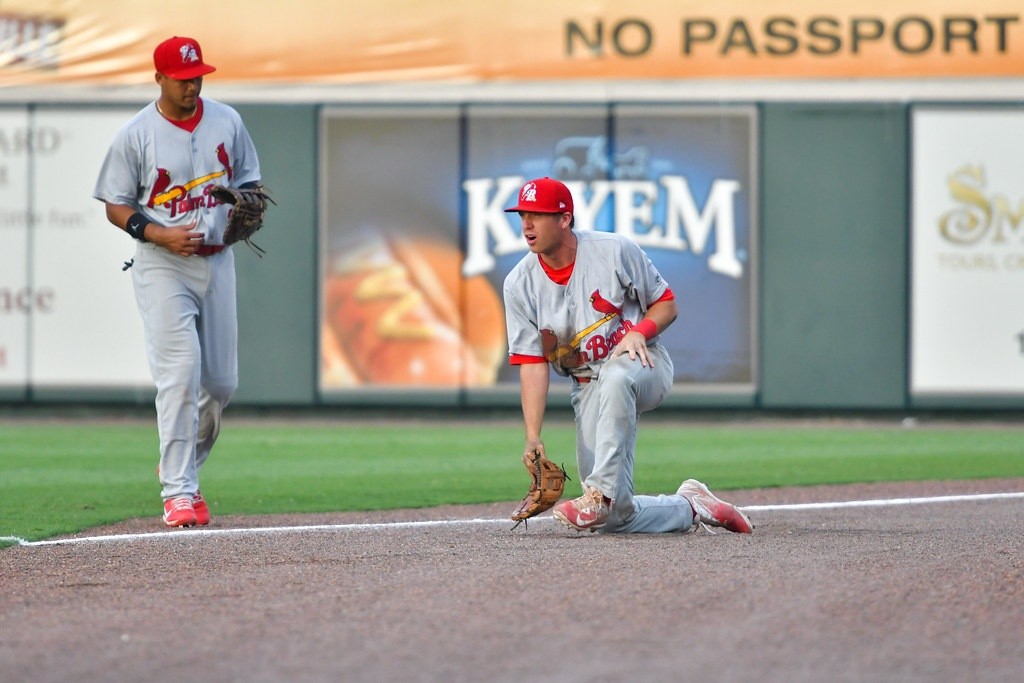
left=157, top=466, right=210, bottom=526
left=552, top=482, right=609, bottom=533
left=163, top=497, right=196, bottom=528
left=676, top=479, right=755, bottom=535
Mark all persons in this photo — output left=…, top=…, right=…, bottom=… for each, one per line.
left=501, top=177, right=756, bottom=537
left=93, top=36, right=258, bottom=528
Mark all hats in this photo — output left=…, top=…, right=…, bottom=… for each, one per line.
left=504, top=177, right=573, bottom=214
left=153, top=36, right=216, bottom=80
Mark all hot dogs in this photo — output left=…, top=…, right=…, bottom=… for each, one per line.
left=322, top=237, right=506, bottom=392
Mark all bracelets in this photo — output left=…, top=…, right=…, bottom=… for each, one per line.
left=125, top=213, right=151, bottom=244
left=629, top=319, right=657, bottom=339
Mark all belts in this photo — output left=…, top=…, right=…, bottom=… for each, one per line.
left=194, top=245, right=224, bottom=255
left=575, top=377, right=591, bottom=382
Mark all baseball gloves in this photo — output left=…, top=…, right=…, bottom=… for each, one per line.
left=510, top=449, right=566, bottom=521
left=207, top=184, right=268, bottom=246
